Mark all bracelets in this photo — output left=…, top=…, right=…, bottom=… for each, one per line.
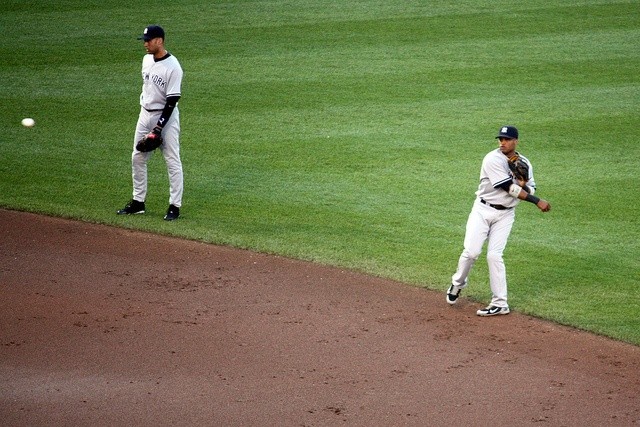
left=525, top=194, right=540, bottom=205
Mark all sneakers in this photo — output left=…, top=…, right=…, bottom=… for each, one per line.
left=476, top=305, right=511, bottom=317
left=163, top=204, right=180, bottom=221
left=446, top=284, right=461, bottom=305
left=117, top=201, right=145, bottom=215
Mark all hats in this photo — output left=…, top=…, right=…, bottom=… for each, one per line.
left=495, top=126, right=518, bottom=139
left=137, top=26, right=165, bottom=40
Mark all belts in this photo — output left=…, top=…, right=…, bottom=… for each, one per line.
left=142, top=107, right=164, bottom=112
left=481, top=199, right=513, bottom=210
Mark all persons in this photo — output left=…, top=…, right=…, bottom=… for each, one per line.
left=116, top=26, right=184, bottom=220
left=446, top=126, right=551, bottom=317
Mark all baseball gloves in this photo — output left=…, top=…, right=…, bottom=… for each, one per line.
left=508, top=154, right=529, bottom=181
left=137, top=133, right=163, bottom=153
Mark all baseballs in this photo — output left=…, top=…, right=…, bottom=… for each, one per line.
left=22, top=117, right=34, bottom=127
